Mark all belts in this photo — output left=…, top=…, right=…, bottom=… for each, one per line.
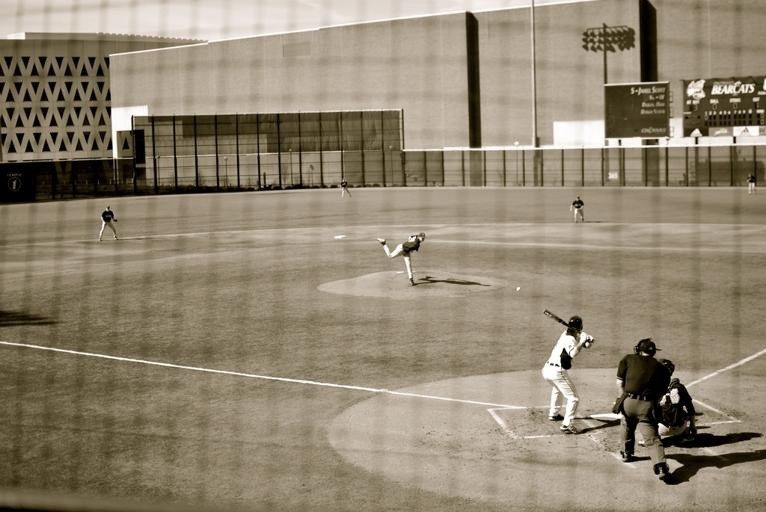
left=627, top=391, right=652, bottom=401
left=550, top=363, right=559, bottom=366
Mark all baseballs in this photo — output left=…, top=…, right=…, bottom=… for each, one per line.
left=516, top=287, right=520, bottom=291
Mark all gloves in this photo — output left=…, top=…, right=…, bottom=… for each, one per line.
left=580, top=332, right=594, bottom=345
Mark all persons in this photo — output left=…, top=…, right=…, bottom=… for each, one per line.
left=745, top=173, right=756, bottom=197
left=637, top=378, right=698, bottom=448
left=377, top=232, right=425, bottom=286
left=615, top=338, right=674, bottom=482
left=541, top=316, right=594, bottom=435
left=340, top=177, right=352, bottom=198
left=570, top=195, right=584, bottom=223
left=97, top=205, right=119, bottom=242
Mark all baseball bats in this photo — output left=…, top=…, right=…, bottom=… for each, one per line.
left=542, top=310, right=581, bottom=335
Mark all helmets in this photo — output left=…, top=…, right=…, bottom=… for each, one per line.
left=657, top=358, right=676, bottom=376
left=569, top=316, right=582, bottom=330
left=633, top=338, right=657, bottom=356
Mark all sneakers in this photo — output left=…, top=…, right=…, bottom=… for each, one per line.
left=410, top=280, right=415, bottom=286
left=376, top=237, right=386, bottom=245
left=548, top=414, right=564, bottom=420
left=560, top=424, right=580, bottom=434
left=620, top=440, right=669, bottom=480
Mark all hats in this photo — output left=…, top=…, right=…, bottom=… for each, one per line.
left=419, top=233, right=425, bottom=237
